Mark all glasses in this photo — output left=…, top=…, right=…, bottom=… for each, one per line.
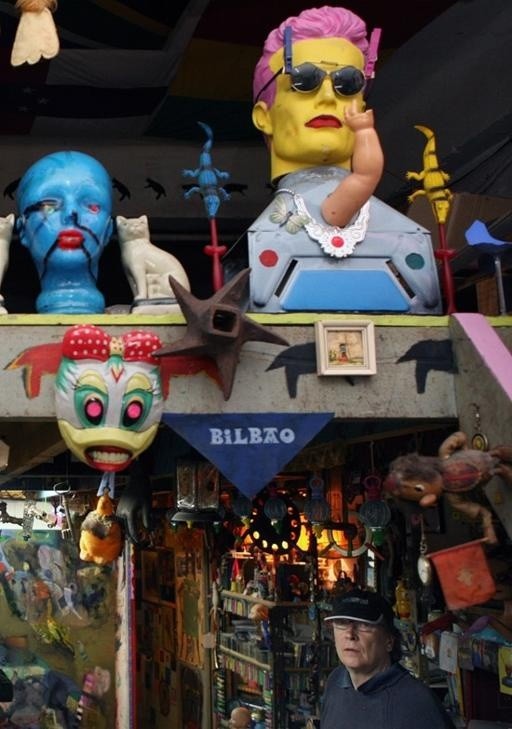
left=332, top=621, right=371, bottom=633
left=255, top=61, right=367, bottom=100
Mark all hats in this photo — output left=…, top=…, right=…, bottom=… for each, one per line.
left=324, top=590, right=396, bottom=625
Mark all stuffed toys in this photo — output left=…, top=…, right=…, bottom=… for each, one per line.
left=77, top=493, right=121, bottom=565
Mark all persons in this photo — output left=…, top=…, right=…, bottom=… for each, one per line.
left=386, top=432, right=512, bottom=545
left=16, top=150, right=114, bottom=313
left=251, top=7, right=384, bottom=228
left=322, top=592, right=452, bottom=729
left=228, top=707, right=252, bottom=728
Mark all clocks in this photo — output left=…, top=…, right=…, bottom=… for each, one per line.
left=417, top=555, right=431, bottom=586
left=314, top=318, right=377, bottom=379
